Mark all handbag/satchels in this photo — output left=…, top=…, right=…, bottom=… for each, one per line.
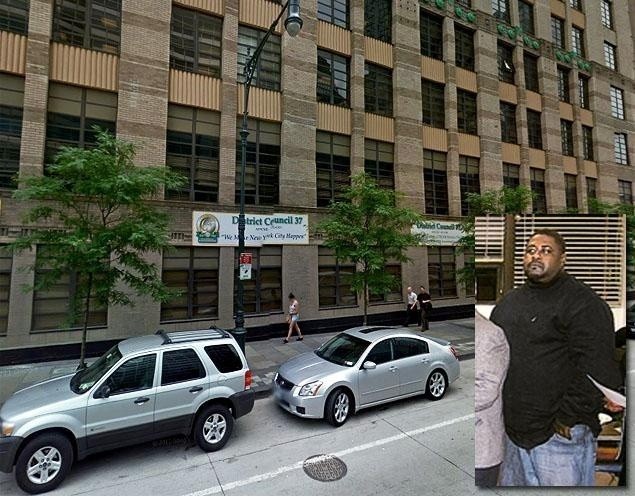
left=425, top=302, right=432, bottom=312
left=285, top=315, right=291, bottom=324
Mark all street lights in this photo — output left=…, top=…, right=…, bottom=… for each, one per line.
left=235, top=0, right=302, bottom=357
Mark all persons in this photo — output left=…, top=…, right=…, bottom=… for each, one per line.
left=602, top=327, right=635, bottom=412
left=282, top=292, right=303, bottom=343
left=402, top=286, right=432, bottom=332
left=475, top=308, right=511, bottom=486
left=489, top=228, right=616, bottom=486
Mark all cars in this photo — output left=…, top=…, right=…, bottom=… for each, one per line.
left=317, top=271, right=382, bottom=303
left=269, top=324, right=462, bottom=429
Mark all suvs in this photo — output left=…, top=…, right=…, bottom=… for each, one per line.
left=193, top=282, right=281, bottom=314
left=0, top=326, right=256, bottom=496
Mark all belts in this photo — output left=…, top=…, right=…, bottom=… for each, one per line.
left=289, top=313, right=298, bottom=315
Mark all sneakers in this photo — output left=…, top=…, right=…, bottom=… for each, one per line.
left=282, top=339, right=289, bottom=344
left=402, top=323, right=429, bottom=332
left=296, top=336, right=304, bottom=341
